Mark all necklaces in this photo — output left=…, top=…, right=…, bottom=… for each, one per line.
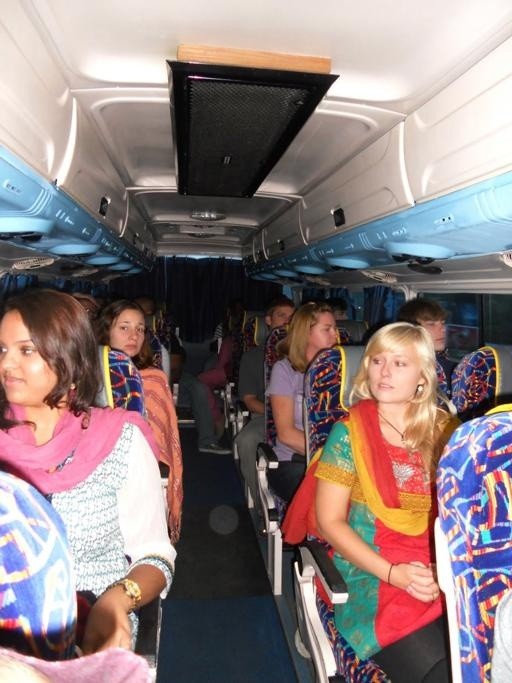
left=367, top=398, right=416, bottom=444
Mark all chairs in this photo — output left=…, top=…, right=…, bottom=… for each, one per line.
left=452, top=345, right=512, bottom=422
left=256, top=323, right=361, bottom=596
left=91, top=346, right=162, bottom=683
left=231, top=312, right=267, bottom=458
left=291, top=346, right=462, bottom=683
left=433, top=411, right=511, bottom=683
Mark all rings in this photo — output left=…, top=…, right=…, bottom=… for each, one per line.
left=432, top=593, right=440, bottom=600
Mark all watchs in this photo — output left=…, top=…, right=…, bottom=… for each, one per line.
left=103, top=579, right=141, bottom=615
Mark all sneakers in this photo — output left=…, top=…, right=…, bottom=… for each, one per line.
left=198, top=442, right=233, bottom=455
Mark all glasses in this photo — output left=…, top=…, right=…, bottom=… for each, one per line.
left=271, top=311, right=294, bottom=322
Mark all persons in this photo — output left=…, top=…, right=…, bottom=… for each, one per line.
left=90, top=299, right=183, bottom=545
left=136, top=298, right=156, bottom=314
left=171, top=369, right=232, bottom=454
left=235, top=296, right=297, bottom=531
left=398, top=298, right=459, bottom=393
left=1, top=289, right=178, bottom=657
left=267, top=302, right=340, bottom=503
left=314, top=322, right=465, bottom=683
left=197, top=333, right=235, bottom=437
left=71, top=292, right=99, bottom=320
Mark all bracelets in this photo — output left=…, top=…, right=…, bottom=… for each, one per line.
left=388, top=563, right=395, bottom=584
